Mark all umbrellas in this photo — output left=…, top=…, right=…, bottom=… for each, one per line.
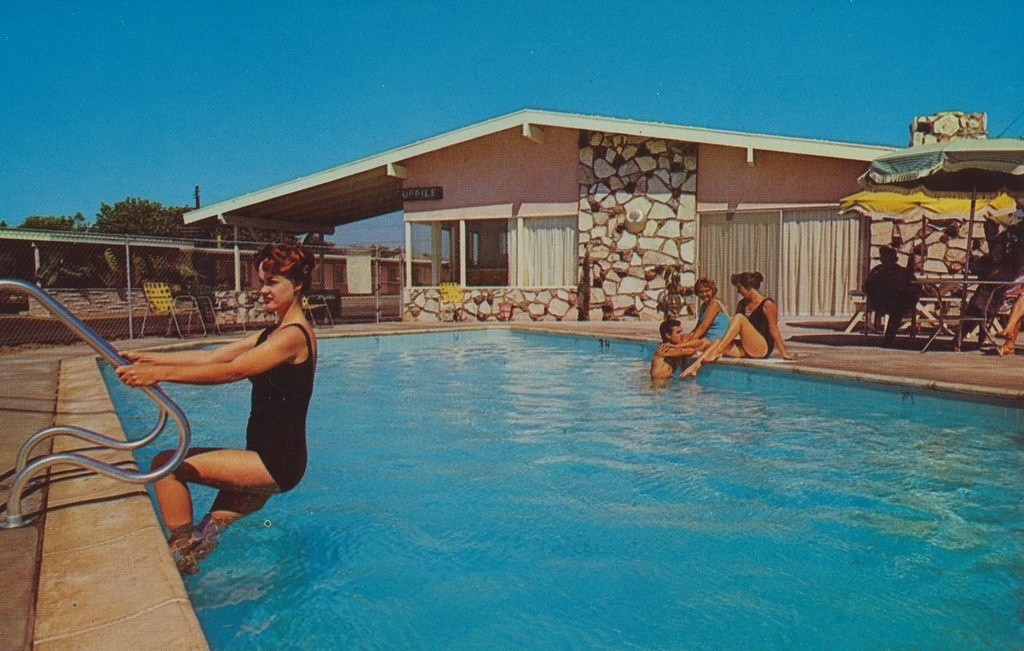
left=857, top=136, right=1024, bottom=351
left=837, top=185, right=1017, bottom=334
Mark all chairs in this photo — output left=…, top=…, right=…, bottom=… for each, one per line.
left=300, top=293, right=336, bottom=331
left=138, top=278, right=208, bottom=341
left=846, top=272, right=1022, bottom=356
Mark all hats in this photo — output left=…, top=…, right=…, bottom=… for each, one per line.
left=256, top=242, right=315, bottom=293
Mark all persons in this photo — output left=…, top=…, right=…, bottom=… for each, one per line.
left=648, top=317, right=711, bottom=391
left=680, top=270, right=797, bottom=379
left=114, top=242, right=318, bottom=574
left=679, top=277, right=731, bottom=350
left=860, top=214, right=1024, bottom=354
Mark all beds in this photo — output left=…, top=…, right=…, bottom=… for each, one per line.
left=187, top=281, right=247, bottom=336
